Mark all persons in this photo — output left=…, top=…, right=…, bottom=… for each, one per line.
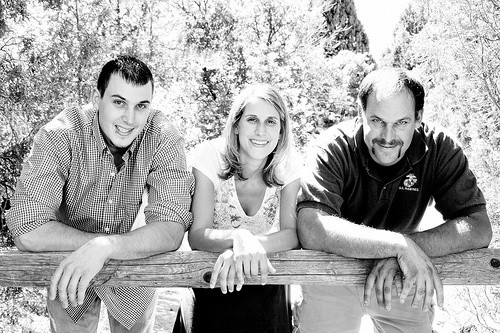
left=6, top=56, right=196, bottom=333
left=293, top=66, right=492, bottom=333
left=186, top=83, right=303, bottom=333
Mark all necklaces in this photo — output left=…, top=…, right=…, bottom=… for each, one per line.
left=237, top=157, right=266, bottom=186
